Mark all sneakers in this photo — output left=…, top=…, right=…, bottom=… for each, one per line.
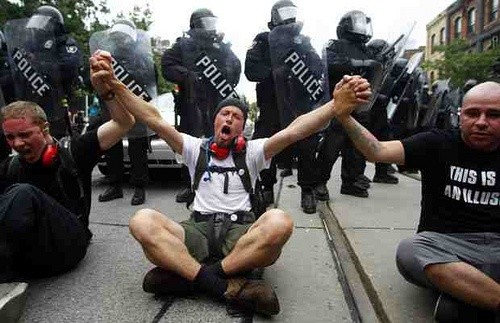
left=131, top=187, right=146, bottom=206
left=280, top=169, right=294, bottom=177
left=224, top=278, right=280, bottom=317
left=99, top=186, right=124, bottom=202
left=340, top=175, right=372, bottom=198
left=372, top=174, right=399, bottom=184
left=0, top=281, right=28, bottom=323
left=175, top=188, right=192, bottom=203
left=261, top=188, right=275, bottom=206
left=142, top=267, right=193, bottom=297
left=299, top=183, right=330, bottom=214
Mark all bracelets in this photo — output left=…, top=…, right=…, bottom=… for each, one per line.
left=95, top=90, right=115, bottom=100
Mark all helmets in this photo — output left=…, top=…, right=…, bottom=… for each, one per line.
left=367, top=39, right=397, bottom=59
left=189, top=9, right=216, bottom=29
left=35, top=5, right=64, bottom=24
left=268, top=1, right=296, bottom=31
left=337, top=11, right=372, bottom=43
left=111, top=18, right=137, bottom=31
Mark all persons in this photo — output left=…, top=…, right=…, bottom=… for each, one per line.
left=315, top=11, right=373, bottom=202
left=91, top=18, right=158, bottom=205
left=342, top=30, right=480, bottom=189
left=90, top=49, right=372, bottom=316
left=0, top=6, right=88, bottom=163
left=336, top=74, right=500, bottom=322
left=1, top=50, right=135, bottom=323
left=162, top=8, right=241, bottom=202
left=245, top=1, right=324, bottom=214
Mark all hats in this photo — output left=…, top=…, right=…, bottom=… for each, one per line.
left=212, top=98, right=250, bottom=124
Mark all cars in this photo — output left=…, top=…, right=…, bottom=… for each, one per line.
left=97, top=92, right=255, bottom=186
